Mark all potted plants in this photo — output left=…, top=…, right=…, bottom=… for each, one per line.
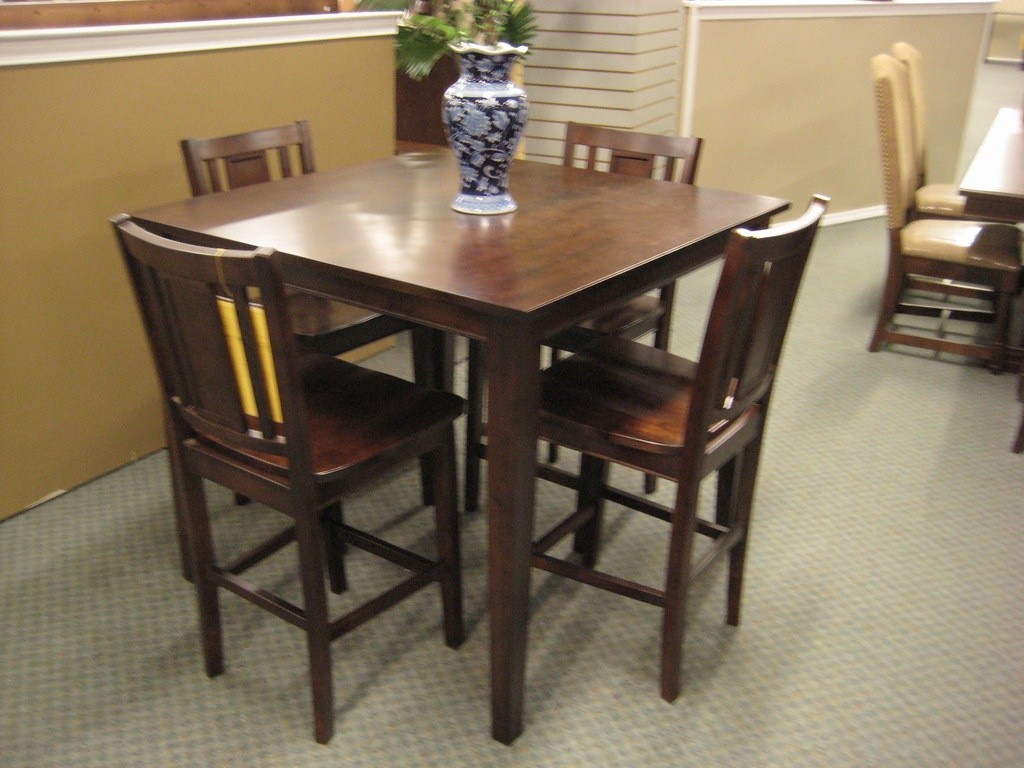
left=352, top=0, right=539, bottom=215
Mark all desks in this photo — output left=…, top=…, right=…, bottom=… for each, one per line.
left=126, top=146, right=795, bottom=744
left=957, top=107, right=1024, bottom=223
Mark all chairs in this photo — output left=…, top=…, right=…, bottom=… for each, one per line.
left=180, top=120, right=436, bottom=509
left=464, top=119, right=704, bottom=515
left=536, top=193, right=832, bottom=702
left=891, top=41, right=1018, bottom=310
left=108, top=211, right=464, bottom=744
left=869, top=53, right=1024, bottom=376
